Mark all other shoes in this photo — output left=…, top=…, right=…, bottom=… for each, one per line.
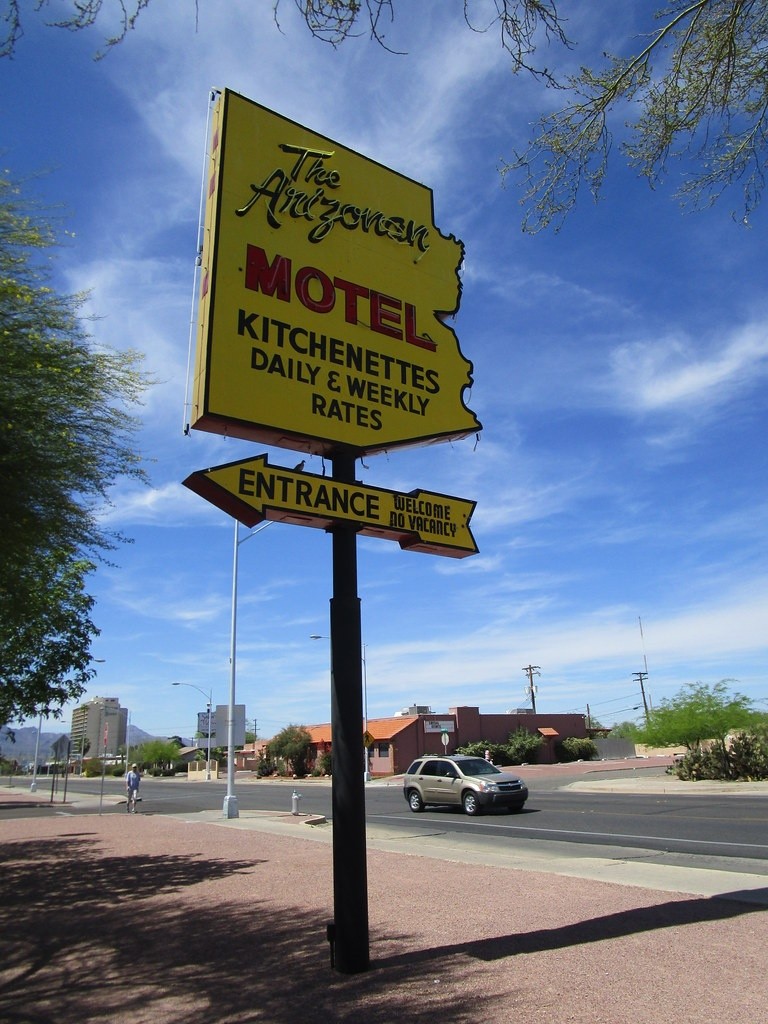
left=132, top=810, right=137, bottom=813
left=126, top=808, right=129, bottom=813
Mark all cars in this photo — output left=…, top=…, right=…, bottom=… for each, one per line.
left=403, top=753, right=531, bottom=816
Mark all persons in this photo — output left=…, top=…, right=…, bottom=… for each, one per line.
left=126, top=763, right=141, bottom=813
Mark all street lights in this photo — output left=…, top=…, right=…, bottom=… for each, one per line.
left=310, top=634, right=372, bottom=780
left=171, top=680, right=215, bottom=778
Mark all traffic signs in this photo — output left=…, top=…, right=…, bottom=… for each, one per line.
left=179, top=451, right=482, bottom=561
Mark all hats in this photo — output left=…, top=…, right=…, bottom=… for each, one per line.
left=132, top=763, right=137, bottom=767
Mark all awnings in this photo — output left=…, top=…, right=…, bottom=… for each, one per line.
left=538, top=727, right=559, bottom=736
